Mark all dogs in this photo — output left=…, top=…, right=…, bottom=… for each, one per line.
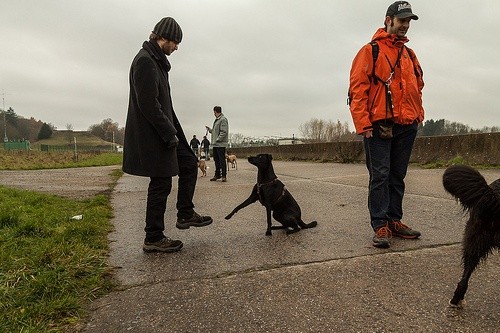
left=442, top=163, right=500, bottom=308
left=225, top=154, right=237, bottom=171
left=224, top=153, right=318, bottom=236
left=197, top=160, right=210, bottom=177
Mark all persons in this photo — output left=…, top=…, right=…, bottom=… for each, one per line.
left=200, top=136, right=211, bottom=161
left=205, top=106, right=229, bottom=182
left=347, top=1, right=424, bottom=249
left=189, top=134, right=200, bottom=162
left=123, top=16, right=213, bottom=252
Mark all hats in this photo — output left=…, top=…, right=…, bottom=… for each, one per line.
left=203, top=136, right=207, bottom=138
left=386, top=1, right=419, bottom=21
left=153, top=15, right=182, bottom=45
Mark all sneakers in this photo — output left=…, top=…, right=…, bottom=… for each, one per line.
left=142, top=210, right=213, bottom=254
left=371, top=218, right=421, bottom=249
left=210, top=175, right=227, bottom=182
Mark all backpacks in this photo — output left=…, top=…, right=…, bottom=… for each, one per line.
left=347, top=39, right=420, bottom=105
